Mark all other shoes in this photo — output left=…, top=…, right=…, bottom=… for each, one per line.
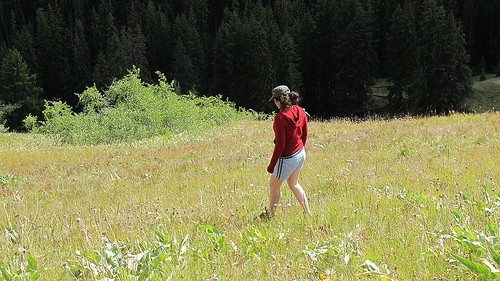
left=255, top=214, right=277, bottom=220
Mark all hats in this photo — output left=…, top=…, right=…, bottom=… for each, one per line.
left=268, top=85, right=289, bottom=102
